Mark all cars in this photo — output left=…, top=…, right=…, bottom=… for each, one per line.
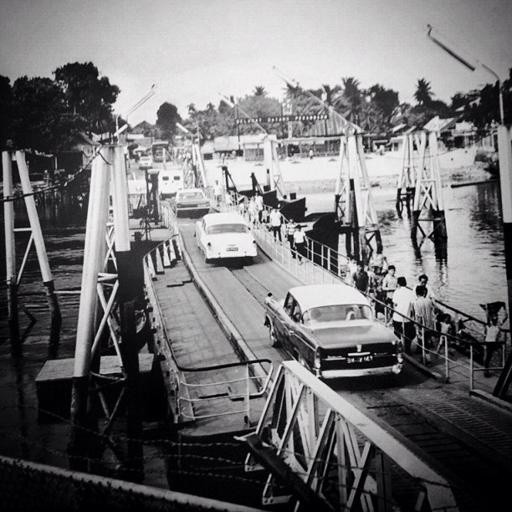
left=139, top=156, right=153, bottom=169
left=147, top=168, right=159, bottom=182
left=195, top=212, right=259, bottom=263
left=265, top=283, right=405, bottom=383
left=173, top=188, right=212, bottom=219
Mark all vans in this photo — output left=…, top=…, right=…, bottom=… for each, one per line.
left=158, top=170, right=186, bottom=199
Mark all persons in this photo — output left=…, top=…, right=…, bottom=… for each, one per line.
left=346, top=245, right=454, bottom=366
left=213, top=180, right=308, bottom=265
left=483, top=302, right=508, bottom=377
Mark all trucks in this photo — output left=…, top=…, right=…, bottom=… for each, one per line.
left=152, top=141, right=170, bottom=162
